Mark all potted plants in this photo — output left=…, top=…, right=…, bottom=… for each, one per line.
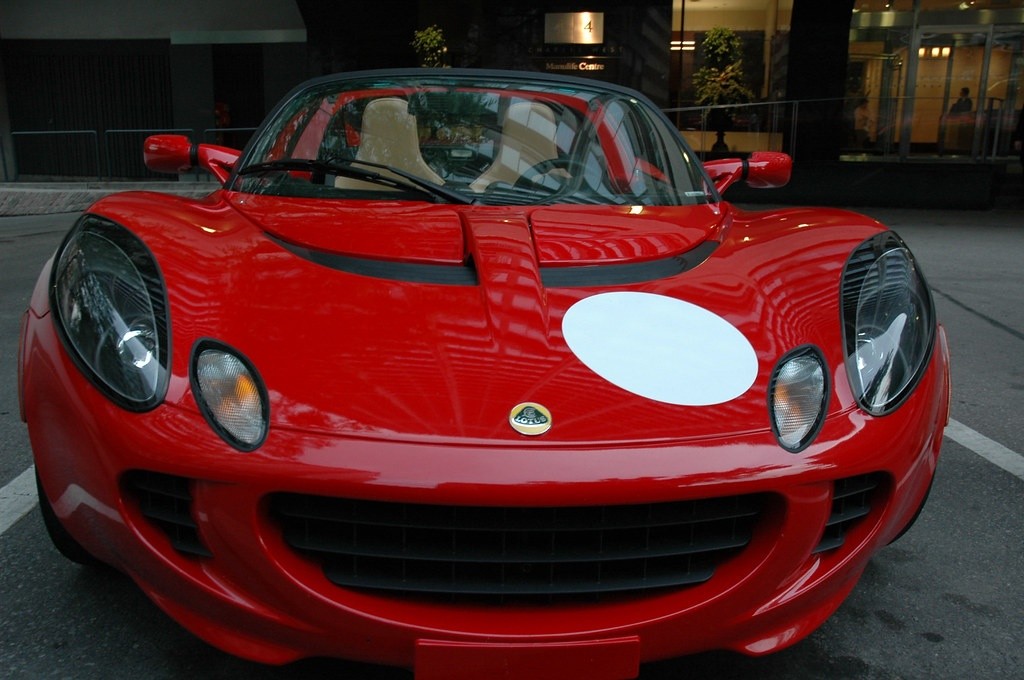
left=691, top=24, right=752, bottom=205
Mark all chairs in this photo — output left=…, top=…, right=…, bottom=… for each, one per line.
left=334, top=98, right=447, bottom=194
left=469, top=101, right=576, bottom=193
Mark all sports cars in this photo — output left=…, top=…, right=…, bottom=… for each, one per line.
left=14, top=62, right=954, bottom=680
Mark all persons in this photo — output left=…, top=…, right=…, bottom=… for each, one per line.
left=215, top=87, right=230, bottom=142
left=1014, top=105, right=1024, bottom=166
left=952, top=87, right=972, bottom=113
